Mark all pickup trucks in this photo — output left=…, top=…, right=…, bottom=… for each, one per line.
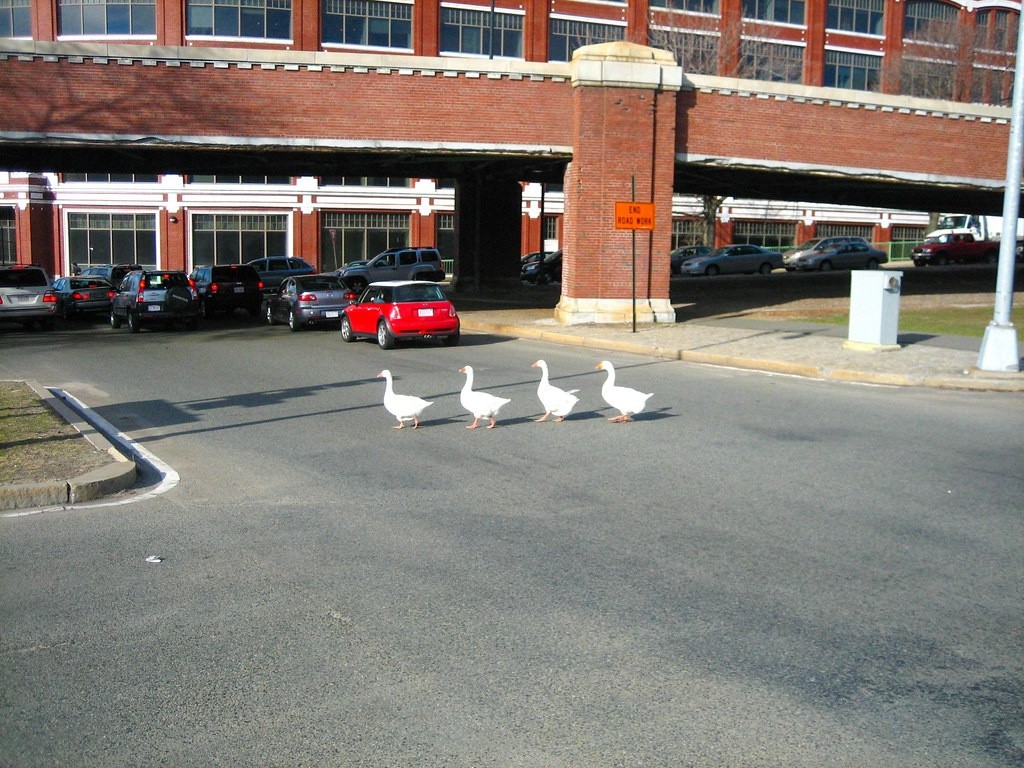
left=909, top=231, right=999, bottom=267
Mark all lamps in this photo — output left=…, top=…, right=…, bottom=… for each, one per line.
left=170, top=217, right=177, bottom=223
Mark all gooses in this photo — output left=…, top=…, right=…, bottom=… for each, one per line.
left=531, top=360, right=579, bottom=422
left=459, top=366, right=511, bottom=428
left=377, top=370, right=434, bottom=429
left=596, top=360, right=654, bottom=423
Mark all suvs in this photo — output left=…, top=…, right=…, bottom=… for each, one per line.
left=75, top=263, right=144, bottom=295
left=330, top=245, right=445, bottom=293
left=0, top=264, right=57, bottom=332
left=244, top=256, right=318, bottom=298
left=191, top=264, right=264, bottom=323
left=110, top=269, right=200, bottom=335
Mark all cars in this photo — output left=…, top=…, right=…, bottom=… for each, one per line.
left=681, top=244, right=783, bottom=276
left=1013, top=235, right=1024, bottom=263
left=521, top=247, right=562, bottom=286
left=782, top=236, right=875, bottom=272
left=339, top=280, right=461, bottom=352
left=521, top=251, right=554, bottom=275
left=265, top=276, right=354, bottom=333
left=52, top=273, right=122, bottom=323
left=670, top=245, right=716, bottom=277
left=801, top=242, right=889, bottom=270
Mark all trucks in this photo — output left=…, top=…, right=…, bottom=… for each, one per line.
left=922, top=214, right=1024, bottom=244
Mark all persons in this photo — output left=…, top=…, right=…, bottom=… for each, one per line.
left=71, top=261, right=81, bottom=276
